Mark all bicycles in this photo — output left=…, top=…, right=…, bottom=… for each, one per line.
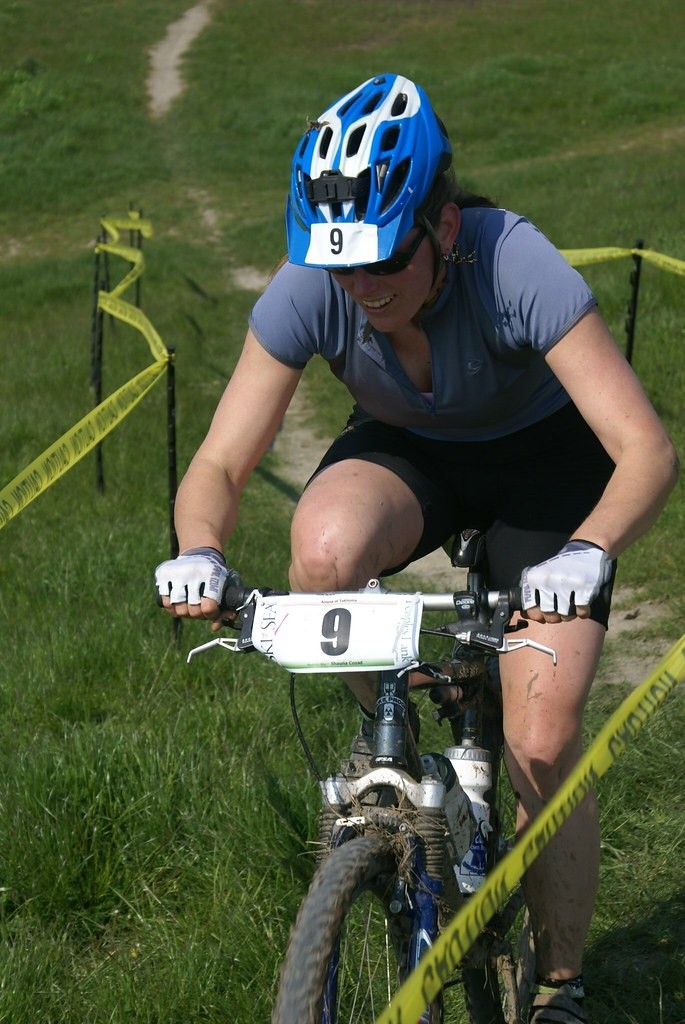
left=184, top=520, right=558, bottom=1023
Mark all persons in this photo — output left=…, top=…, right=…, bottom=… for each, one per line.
left=154, top=72, right=680, bottom=1024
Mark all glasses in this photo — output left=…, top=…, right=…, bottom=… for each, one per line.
left=322, top=210, right=438, bottom=276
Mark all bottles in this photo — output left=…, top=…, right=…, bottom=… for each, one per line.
left=443, top=732, right=492, bottom=893
left=420, top=752, right=476, bottom=864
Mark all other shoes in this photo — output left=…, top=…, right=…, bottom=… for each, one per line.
left=527, top=975, right=586, bottom=1024
left=345, top=701, right=421, bottom=838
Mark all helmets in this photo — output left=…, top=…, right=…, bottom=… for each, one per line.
left=285, top=73, right=452, bottom=267
